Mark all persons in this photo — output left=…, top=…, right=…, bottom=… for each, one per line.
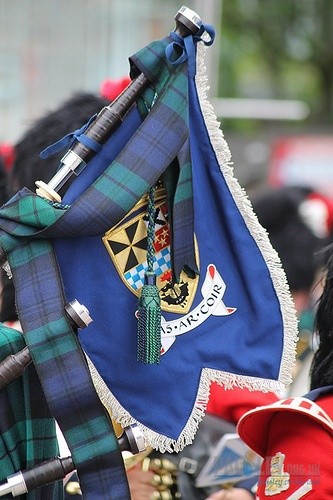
left=1, top=78, right=333, bottom=500
left=236, top=243, right=333, bottom=500
